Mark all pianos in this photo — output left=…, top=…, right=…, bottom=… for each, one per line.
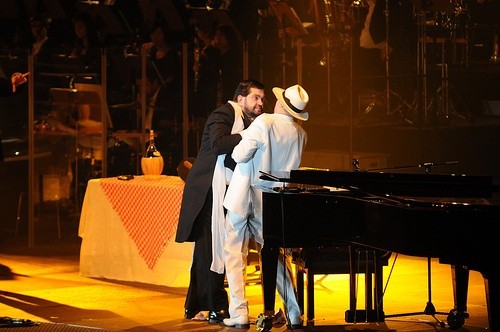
left=259, top=167, right=500, bottom=332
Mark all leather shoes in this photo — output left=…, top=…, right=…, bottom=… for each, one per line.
left=285, top=312, right=301, bottom=328
left=184, top=309, right=201, bottom=319
left=208, top=310, right=231, bottom=324
left=223, top=315, right=250, bottom=329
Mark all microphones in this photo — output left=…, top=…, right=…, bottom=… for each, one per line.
left=352, top=158, right=359, bottom=171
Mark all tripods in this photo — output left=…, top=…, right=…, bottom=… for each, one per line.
left=355, top=0, right=466, bottom=127
left=362, top=161, right=470, bottom=327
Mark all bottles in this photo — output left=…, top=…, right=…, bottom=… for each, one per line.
left=141, top=130, right=164, bottom=176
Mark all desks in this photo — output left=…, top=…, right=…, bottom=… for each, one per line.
left=78, top=174, right=194, bottom=288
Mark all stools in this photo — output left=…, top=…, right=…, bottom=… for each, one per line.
left=292, top=248, right=390, bottom=328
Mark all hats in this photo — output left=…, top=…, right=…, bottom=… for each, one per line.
left=272, top=84, right=309, bottom=121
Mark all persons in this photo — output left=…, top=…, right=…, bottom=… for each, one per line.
left=220, top=86, right=310, bottom=331
left=174, top=81, right=265, bottom=325
left=1, top=0, right=271, bottom=178
left=352, top=0, right=500, bottom=118
